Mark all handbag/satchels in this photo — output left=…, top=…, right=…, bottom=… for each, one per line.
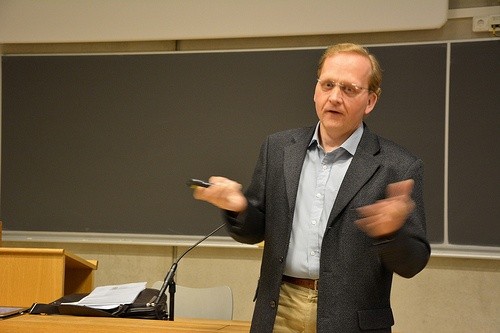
left=29, top=288, right=167, bottom=318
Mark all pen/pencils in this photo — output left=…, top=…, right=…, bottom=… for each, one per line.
left=186, top=177, right=214, bottom=191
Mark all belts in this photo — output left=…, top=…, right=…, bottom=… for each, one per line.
left=281, top=274, right=319, bottom=290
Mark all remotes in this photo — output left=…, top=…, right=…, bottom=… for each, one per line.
left=187, top=179, right=214, bottom=188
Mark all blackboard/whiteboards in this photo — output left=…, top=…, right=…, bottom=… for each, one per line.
left=0, top=37, right=500, bottom=261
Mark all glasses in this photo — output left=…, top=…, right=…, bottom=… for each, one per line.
left=317, top=79, right=371, bottom=97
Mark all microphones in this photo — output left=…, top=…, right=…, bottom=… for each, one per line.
left=154, top=218, right=239, bottom=303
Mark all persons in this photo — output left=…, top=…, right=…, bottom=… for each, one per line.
left=186, top=39, right=431, bottom=333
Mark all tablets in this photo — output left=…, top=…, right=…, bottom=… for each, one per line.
left=0, top=306, right=29, bottom=318
